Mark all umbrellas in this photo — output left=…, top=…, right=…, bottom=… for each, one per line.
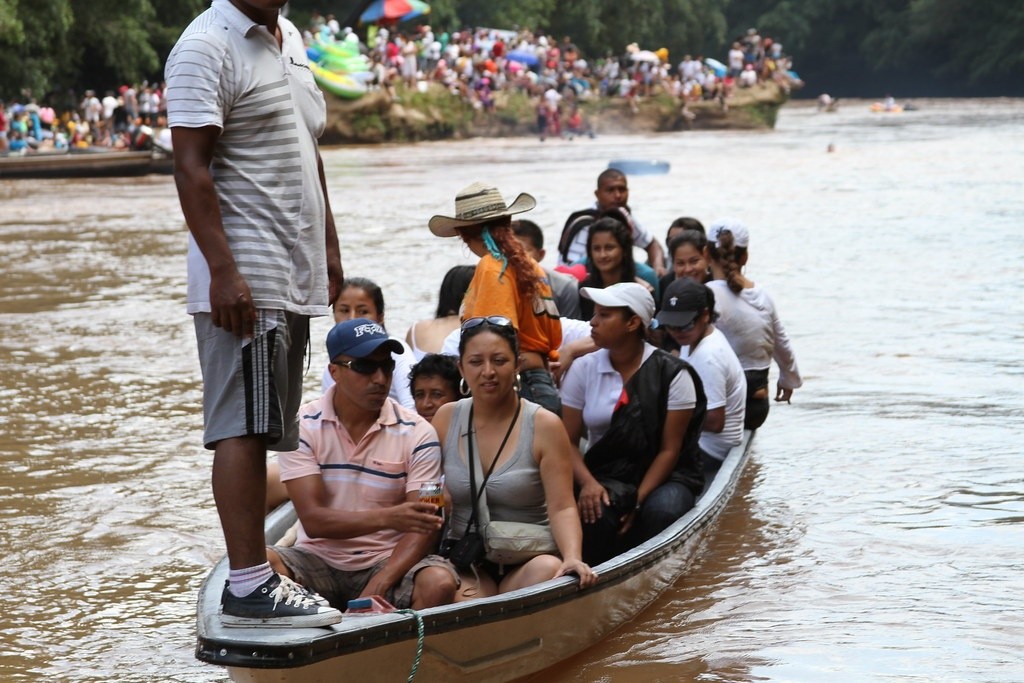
left=359, top=0, right=431, bottom=26
left=504, top=49, right=538, bottom=64
left=630, top=50, right=658, bottom=63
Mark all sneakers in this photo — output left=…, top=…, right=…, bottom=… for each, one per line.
left=218, top=570, right=342, bottom=629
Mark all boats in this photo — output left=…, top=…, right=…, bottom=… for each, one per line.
left=0, top=145, right=174, bottom=179
left=195, top=427, right=758, bottom=682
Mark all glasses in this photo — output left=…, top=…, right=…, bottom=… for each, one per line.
left=459, top=315, right=519, bottom=352
left=664, top=315, right=699, bottom=331
left=332, top=357, right=396, bottom=377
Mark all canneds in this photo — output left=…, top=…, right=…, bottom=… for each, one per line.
left=420, top=481, right=442, bottom=518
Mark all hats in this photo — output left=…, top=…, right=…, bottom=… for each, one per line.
left=451, top=32, right=460, bottom=39
left=579, top=282, right=656, bottom=332
left=428, top=181, right=537, bottom=238
left=706, top=220, right=749, bottom=248
left=654, top=277, right=708, bottom=326
left=326, top=318, right=404, bottom=363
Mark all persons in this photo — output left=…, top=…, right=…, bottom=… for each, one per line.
left=0, top=80, right=168, bottom=151
left=265, top=168, right=803, bottom=610
left=883, top=94, right=895, bottom=112
left=298, top=8, right=806, bottom=141
left=165, top=0, right=344, bottom=628
left=817, top=92, right=831, bottom=111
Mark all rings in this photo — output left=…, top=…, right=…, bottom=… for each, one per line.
left=590, top=571, right=596, bottom=577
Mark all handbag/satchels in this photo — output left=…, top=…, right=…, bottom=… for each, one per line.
left=447, top=532, right=486, bottom=574
left=483, top=519, right=560, bottom=563
